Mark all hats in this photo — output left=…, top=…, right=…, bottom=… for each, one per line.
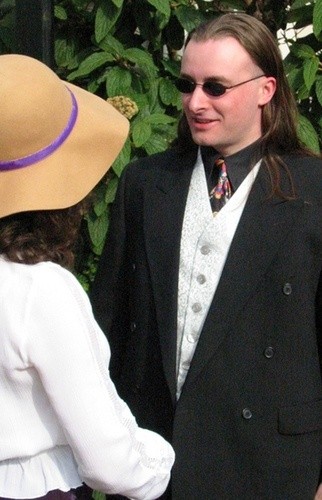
left=1, top=54, right=129, bottom=217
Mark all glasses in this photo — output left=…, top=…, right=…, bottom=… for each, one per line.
left=174, top=75, right=267, bottom=98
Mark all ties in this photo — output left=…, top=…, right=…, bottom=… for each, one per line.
left=209, top=157, right=231, bottom=213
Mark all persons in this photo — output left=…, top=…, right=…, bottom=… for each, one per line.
left=88, top=13, right=322, bottom=500
left=0, top=53, right=175, bottom=500
